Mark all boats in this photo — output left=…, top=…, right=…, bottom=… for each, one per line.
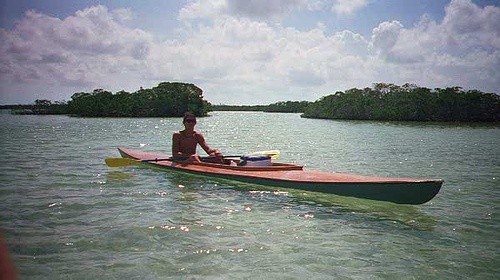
left=117, top=146, right=445, bottom=205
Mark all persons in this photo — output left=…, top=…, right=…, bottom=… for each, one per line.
left=172, top=113, right=224, bottom=166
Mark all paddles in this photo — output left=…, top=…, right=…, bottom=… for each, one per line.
left=105, top=148, right=279, bottom=168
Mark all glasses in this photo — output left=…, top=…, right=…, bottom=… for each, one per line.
left=184, top=118, right=196, bottom=123
